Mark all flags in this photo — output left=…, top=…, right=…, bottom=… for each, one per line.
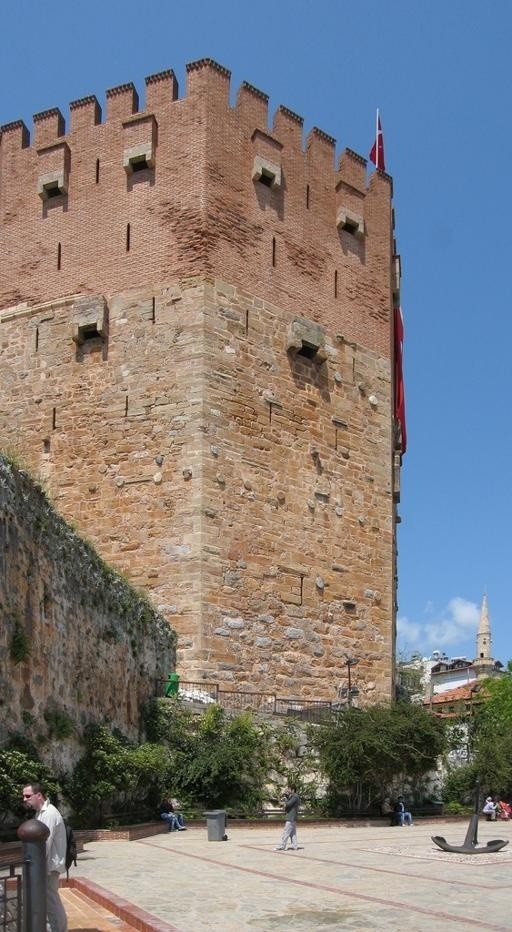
left=369, top=115, right=388, bottom=172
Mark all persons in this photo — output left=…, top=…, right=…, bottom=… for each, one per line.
left=482, top=795, right=495, bottom=821
left=159, top=792, right=186, bottom=832
left=395, top=796, right=414, bottom=827
left=23, top=780, right=70, bottom=931
left=274, top=784, right=300, bottom=850
left=381, top=797, right=400, bottom=826
left=482, top=799, right=498, bottom=821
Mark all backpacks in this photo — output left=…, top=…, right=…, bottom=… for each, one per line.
left=63, top=818, right=77, bottom=869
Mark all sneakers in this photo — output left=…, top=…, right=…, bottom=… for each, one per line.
left=390, top=823, right=414, bottom=827
left=275, top=847, right=296, bottom=851
left=170, top=826, right=186, bottom=832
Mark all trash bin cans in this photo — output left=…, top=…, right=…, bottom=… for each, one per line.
left=203, top=810, right=227, bottom=841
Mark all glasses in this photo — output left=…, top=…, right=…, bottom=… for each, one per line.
left=22, top=793, right=37, bottom=798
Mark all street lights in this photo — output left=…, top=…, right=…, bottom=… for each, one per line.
left=336, top=658, right=363, bottom=713
left=469, top=684, right=483, bottom=721
